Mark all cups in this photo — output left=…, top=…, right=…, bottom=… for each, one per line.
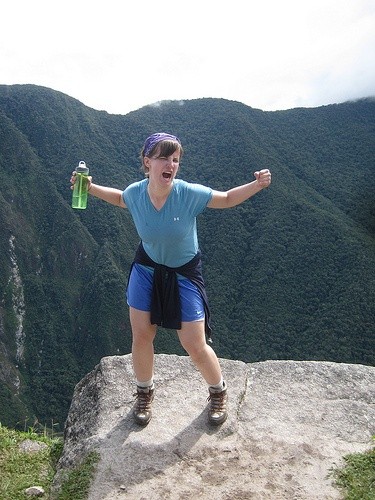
left=71, top=161, right=89, bottom=210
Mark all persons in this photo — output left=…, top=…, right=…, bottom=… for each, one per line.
left=70, top=133, right=271, bottom=424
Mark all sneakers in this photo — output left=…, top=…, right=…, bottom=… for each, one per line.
left=207, top=381, right=227, bottom=425
left=133, top=386, right=155, bottom=425
